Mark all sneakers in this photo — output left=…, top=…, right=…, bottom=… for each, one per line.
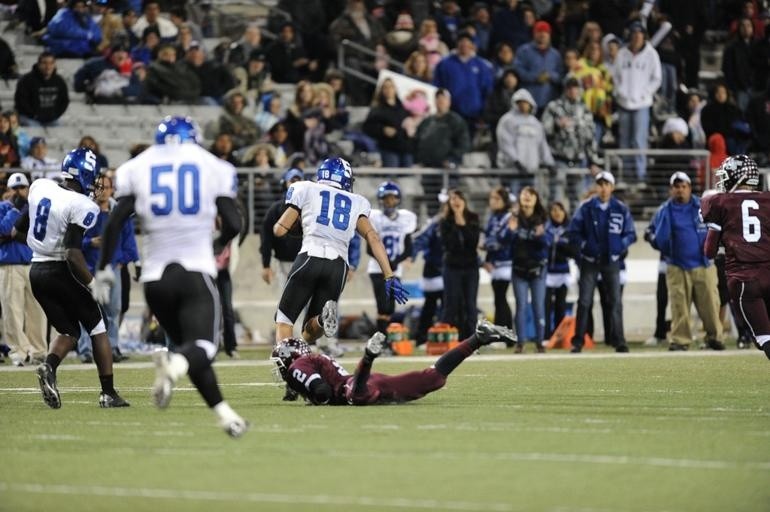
left=216, top=406, right=248, bottom=437
left=149, top=347, right=176, bottom=409
left=515, top=344, right=524, bottom=353
left=322, top=300, right=339, bottom=337
left=537, top=342, right=543, bottom=352
left=475, top=319, right=519, bottom=345
left=37, top=348, right=133, bottom=408
left=365, top=331, right=386, bottom=355
left=643, top=337, right=753, bottom=351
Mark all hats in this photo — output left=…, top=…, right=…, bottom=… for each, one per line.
left=596, top=172, right=615, bottom=184
left=533, top=20, right=551, bottom=33
left=670, top=171, right=692, bottom=187
left=662, top=116, right=689, bottom=136
left=6, top=172, right=28, bottom=189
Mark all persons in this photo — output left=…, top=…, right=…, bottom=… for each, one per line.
left=270, top=317, right=518, bottom=405
left=89, top=116, right=246, bottom=438
left=28, top=148, right=129, bottom=410
left=1, top=2, right=770, bottom=369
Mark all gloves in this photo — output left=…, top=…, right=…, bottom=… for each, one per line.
left=87, top=278, right=110, bottom=305
left=94, top=263, right=116, bottom=285
left=384, top=277, right=410, bottom=305
left=13, top=215, right=29, bottom=232
left=13, top=197, right=25, bottom=210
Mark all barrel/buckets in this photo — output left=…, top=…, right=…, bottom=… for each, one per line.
left=386, top=321, right=418, bottom=354
left=427, top=319, right=461, bottom=356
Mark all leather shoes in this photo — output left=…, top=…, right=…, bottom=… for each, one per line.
left=617, top=345, right=628, bottom=352
left=571, top=346, right=581, bottom=353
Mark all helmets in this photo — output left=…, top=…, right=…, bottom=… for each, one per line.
left=156, top=114, right=197, bottom=146
left=714, top=154, right=761, bottom=193
left=378, top=182, right=403, bottom=211
left=284, top=170, right=304, bottom=182
left=269, top=336, right=312, bottom=380
left=316, top=156, right=354, bottom=193
left=61, top=146, right=108, bottom=200
